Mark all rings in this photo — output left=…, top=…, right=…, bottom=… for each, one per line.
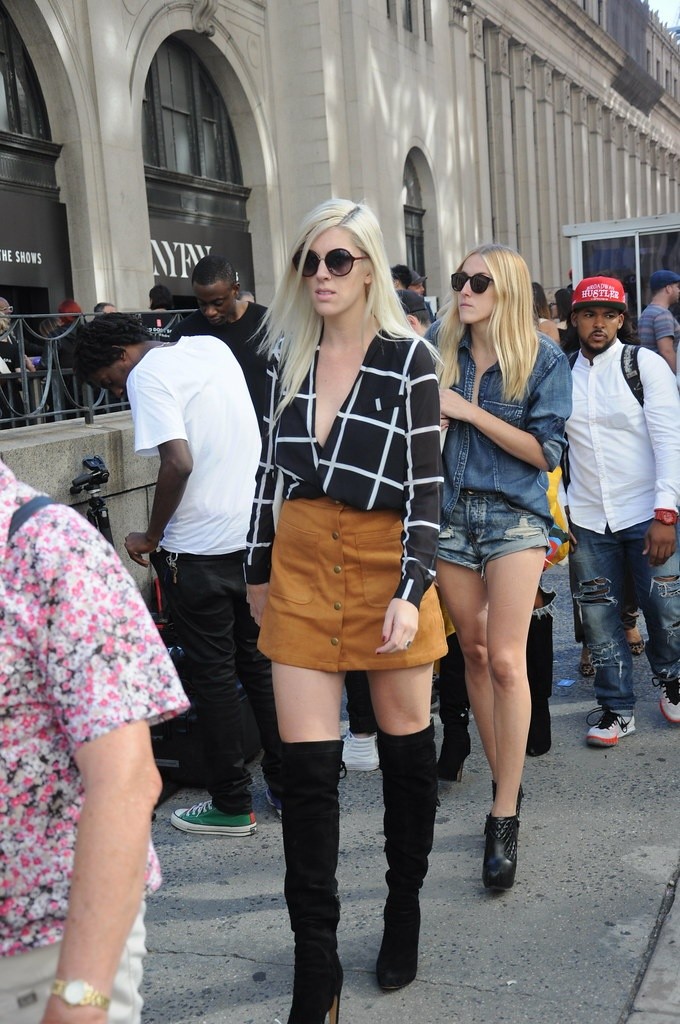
left=405, top=641, right=412, bottom=648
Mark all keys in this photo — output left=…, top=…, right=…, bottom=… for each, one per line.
left=171, top=564, right=178, bottom=584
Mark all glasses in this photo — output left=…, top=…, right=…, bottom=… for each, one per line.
left=0, top=307, right=13, bottom=311
left=451, top=272, right=494, bottom=294
left=293, top=248, right=368, bottom=276
left=195, top=283, right=234, bottom=309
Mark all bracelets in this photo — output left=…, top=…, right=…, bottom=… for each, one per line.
left=146, top=531, right=159, bottom=547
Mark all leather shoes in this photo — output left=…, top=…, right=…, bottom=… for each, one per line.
left=482, top=816, right=517, bottom=890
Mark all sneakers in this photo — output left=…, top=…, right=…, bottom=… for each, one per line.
left=660, top=679, right=680, bottom=722
left=171, top=800, right=257, bottom=837
left=587, top=711, right=636, bottom=747
left=341, top=732, right=380, bottom=771
left=266, top=789, right=282, bottom=820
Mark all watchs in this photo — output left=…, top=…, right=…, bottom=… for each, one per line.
left=51, top=978, right=110, bottom=1010
left=653, top=511, right=677, bottom=526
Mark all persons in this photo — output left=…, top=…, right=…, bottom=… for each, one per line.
left=0, top=256, right=680, bottom=892
left=242, top=197, right=451, bottom=1024
left=0, top=458, right=194, bottom=1024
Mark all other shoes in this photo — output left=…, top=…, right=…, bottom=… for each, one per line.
left=623, top=626, right=644, bottom=655
left=492, top=780, right=520, bottom=828
left=431, top=696, right=439, bottom=713
left=581, top=646, right=593, bottom=675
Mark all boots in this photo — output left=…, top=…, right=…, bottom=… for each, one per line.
left=526, top=614, right=558, bottom=756
left=376, top=717, right=438, bottom=989
left=437, top=633, right=470, bottom=782
left=279, top=740, right=345, bottom=1024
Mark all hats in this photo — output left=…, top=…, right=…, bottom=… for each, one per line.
left=59, top=300, right=81, bottom=323
left=572, top=276, right=626, bottom=310
left=410, top=271, right=427, bottom=285
left=650, top=270, right=680, bottom=289
left=397, top=290, right=427, bottom=314
